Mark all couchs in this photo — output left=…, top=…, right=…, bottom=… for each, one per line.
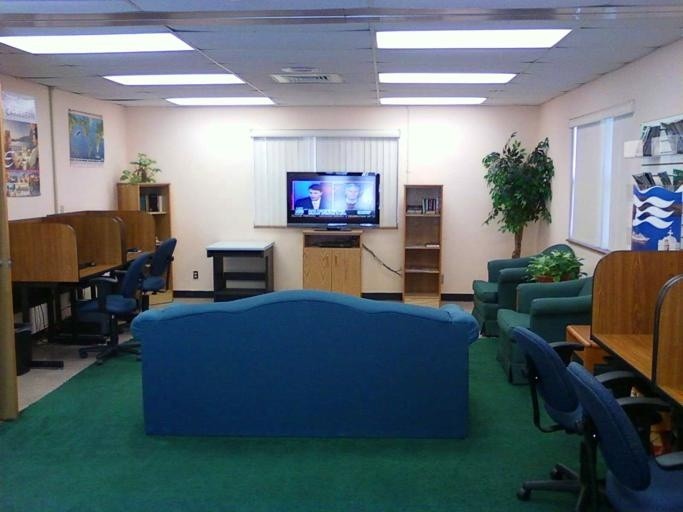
left=472, top=244, right=579, bottom=336
left=497, top=270, right=593, bottom=385
left=130, top=290, right=479, bottom=436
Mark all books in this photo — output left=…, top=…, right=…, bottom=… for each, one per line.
left=141, top=194, right=163, bottom=212
left=654, top=171, right=671, bottom=188
left=407, top=198, right=439, bottom=214
left=633, top=173, right=655, bottom=191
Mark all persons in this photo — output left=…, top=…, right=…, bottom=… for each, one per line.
left=294, top=184, right=330, bottom=215
left=334, top=184, right=371, bottom=214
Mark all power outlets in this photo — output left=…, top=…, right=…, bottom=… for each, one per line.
left=193, top=271, right=198, bottom=279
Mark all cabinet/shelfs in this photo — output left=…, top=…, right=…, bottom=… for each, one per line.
left=117, top=183, right=173, bottom=304
left=402, top=185, right=442, bottom=308
left=302, top=230, right=363, bottom=298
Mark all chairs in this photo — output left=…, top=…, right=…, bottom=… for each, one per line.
left=119, top=238, right=176, bottom=362
left=511, top=319, right=671, bottom=512
left=567, top=362, right=683, bottom=512
left=71, top=254, right=153, bottom=364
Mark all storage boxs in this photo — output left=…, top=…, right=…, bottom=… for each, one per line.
left=15, top=324, right=33, bottom=375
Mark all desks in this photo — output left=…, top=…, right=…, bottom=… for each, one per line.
left=566, top=326, right=610, bottom=369
left=206, top=240, right=274, bottom=303
left=8, top=215, right=119, bottom=367
left=591, top=251, right=683, bottom=408
left=47, top=211, right=155, bottom=331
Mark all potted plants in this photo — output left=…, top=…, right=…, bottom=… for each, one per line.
left=520, top=249, right=588, bottom=282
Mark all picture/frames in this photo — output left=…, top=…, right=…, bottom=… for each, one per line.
left=68, top=109, right=104, bottom=163
left=640, top=113, right=683, bottom=165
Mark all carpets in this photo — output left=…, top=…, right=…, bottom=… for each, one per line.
left=0, top=338, right=617, bottom=512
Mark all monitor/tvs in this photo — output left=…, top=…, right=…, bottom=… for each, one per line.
left=286, top=171, right=380, bottom=231
